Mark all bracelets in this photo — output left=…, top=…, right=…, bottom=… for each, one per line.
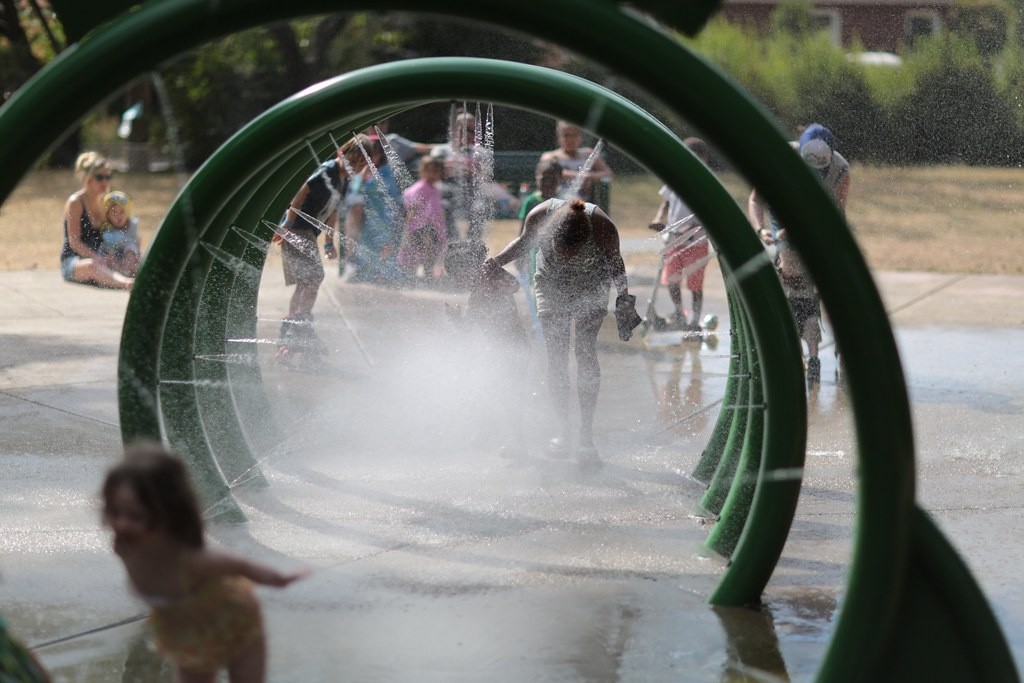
left=757, top=227, right=764, bottom=235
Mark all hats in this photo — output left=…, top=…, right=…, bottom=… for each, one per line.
left=102, top=191, right=133, bottom=218
left=799, top=124, right=835, bottom=179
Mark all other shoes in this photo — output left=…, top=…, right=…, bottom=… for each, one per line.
left=807, top=359, right=820, bottom=374
left=275, top=317, right=327, bottom=347
left=576, top=442, right=597, bottom=460
left=548, top=436, right=571, bottom=451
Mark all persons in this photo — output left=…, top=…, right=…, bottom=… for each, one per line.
left=96, top=191, right=143, bottom=277
left=275, top=111, right=852, bottom=475
left=101, top=446, right=267, bottom=683
left=61, top=151, right=144, bottom=291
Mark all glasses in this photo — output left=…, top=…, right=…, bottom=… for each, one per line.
left=106, top=194, right=127, bottom=205
left=94, top=174, right=111, bottom=181
